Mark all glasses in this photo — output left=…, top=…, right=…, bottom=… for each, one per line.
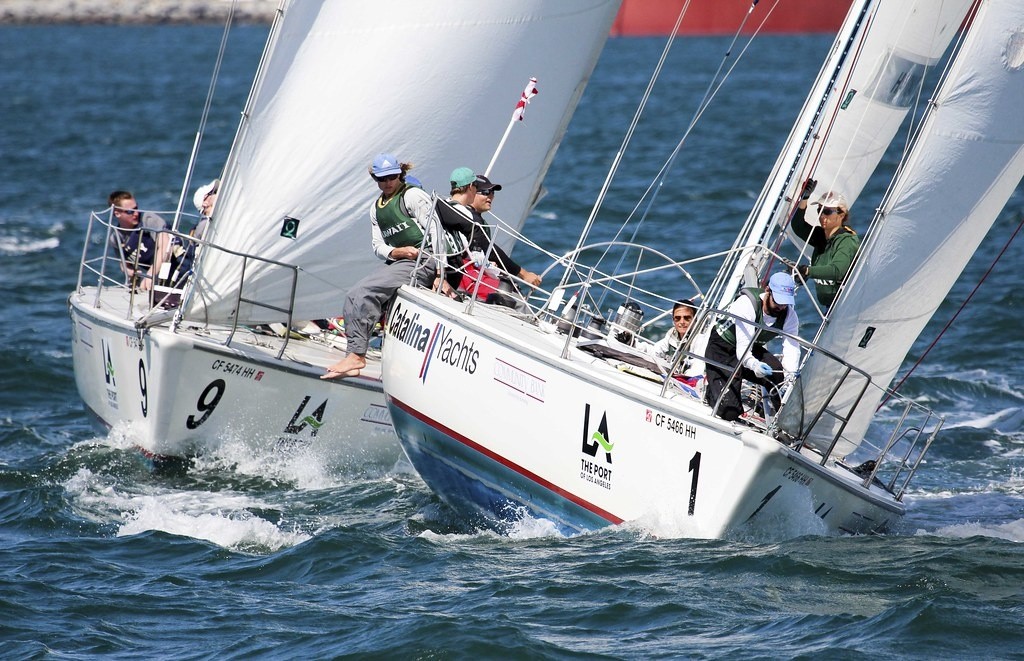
left=117, top=206, right=137, bottom=214
left=818, top=208, right=840, bottom=214
left=472, top=183, right=478, bottom=188
left=204, top=191, right=216, bottom=201
left=377, top=174, right=398, bottom=182
left=478, top=189, right=494, bottom=194
left=673, top=314, right=692, bottom=323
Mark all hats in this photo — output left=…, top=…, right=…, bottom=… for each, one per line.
left=475, top=175, right=501, bottom=191
left=193, top=179, right=220, bottom=213
left=371, top=153, right=402, bottom=177
left=811, top=192, right=847, bottom=214
left=768, top=272, right=795, bottom=305
left=449, top=167, right=486, bottom=189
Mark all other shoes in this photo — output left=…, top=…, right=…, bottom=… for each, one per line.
left=701, top=375, right=710, bottom=406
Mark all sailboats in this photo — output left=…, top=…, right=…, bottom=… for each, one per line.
left=368, top=0, right=1024, bottom=543
left=64, top=0, right=623, bottom=476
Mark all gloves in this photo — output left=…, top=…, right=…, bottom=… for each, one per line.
left=753, top=362, right=773, bottom=378
left=801, top=179, right=817, bottom=199
left=795, top=265, right=807, bottom=282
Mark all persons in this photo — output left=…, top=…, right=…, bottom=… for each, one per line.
left=705, top=272, right=805, bottom=421
left=317, top=151, right=459, bottom=381
left=188, top=178, right=331, bottom=337
left=645, top=299, right=706, bottom=401
left=431, top=167, right=545, bottom=291
left=108, top=191, right=192, bottom=308
left=786, top=175, right=862, bottom=304
